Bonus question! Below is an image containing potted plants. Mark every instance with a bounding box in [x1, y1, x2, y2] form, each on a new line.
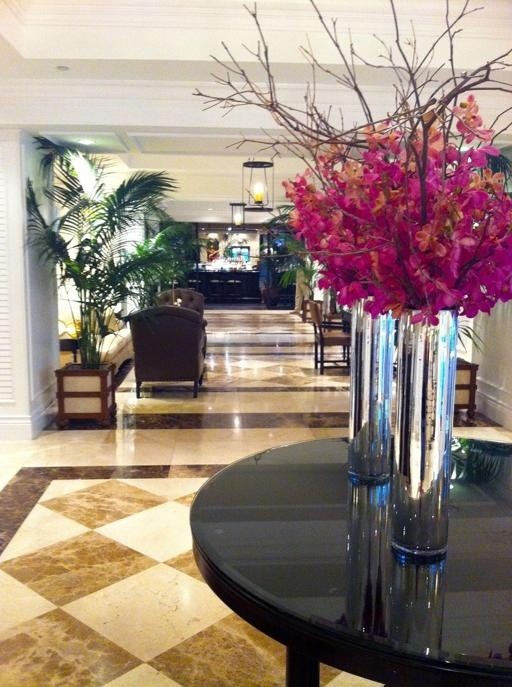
[22, 134, 207, 430]
[452, 356, 478, 420]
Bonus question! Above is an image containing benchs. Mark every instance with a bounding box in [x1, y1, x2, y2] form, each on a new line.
[69, 326, 131, 376]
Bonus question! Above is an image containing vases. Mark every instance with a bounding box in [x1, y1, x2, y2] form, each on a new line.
[347, 293, 459, 564]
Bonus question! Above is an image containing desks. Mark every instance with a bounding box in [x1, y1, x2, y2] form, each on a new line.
[191, 436, 509, 686]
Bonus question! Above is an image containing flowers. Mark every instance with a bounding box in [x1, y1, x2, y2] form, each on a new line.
[287, 96, 512, 321]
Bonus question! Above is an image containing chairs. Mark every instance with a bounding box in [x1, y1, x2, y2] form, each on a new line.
[306, 300, 353, 372]
[127, 289, 207, 399]
